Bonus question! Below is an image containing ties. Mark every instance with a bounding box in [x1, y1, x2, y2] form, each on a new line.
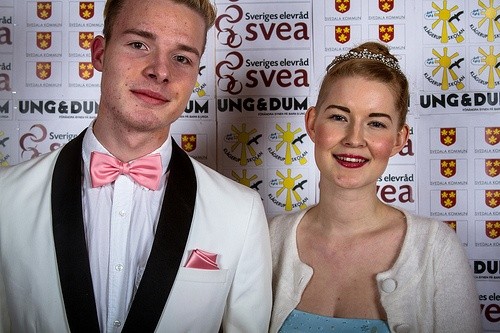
[89, 151, 162, 191]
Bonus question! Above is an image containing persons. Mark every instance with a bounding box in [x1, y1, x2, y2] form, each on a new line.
[261, 42, 481, 333]
[0, 0, 275, 333]
